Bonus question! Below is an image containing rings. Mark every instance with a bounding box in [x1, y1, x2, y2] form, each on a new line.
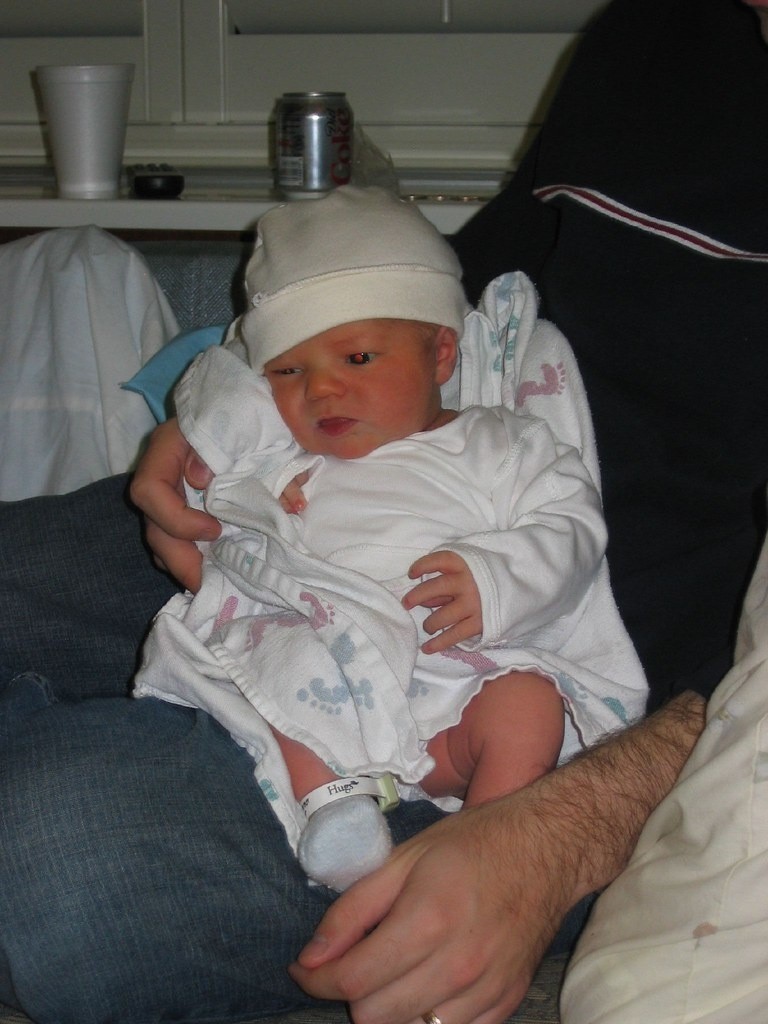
[421, 1011, 441, 1024]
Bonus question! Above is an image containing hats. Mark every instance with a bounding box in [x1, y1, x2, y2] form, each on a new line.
[240, 181, 469, 376]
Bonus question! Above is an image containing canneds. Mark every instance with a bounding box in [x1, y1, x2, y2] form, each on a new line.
[273, 91, 353, 200]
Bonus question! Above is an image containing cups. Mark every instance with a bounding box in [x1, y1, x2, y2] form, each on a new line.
[30, 61, 132, 200]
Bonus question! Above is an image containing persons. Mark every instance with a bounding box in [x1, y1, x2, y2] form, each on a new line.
[134, 181, 650, 886]
[0, 0, 768, 1024]
